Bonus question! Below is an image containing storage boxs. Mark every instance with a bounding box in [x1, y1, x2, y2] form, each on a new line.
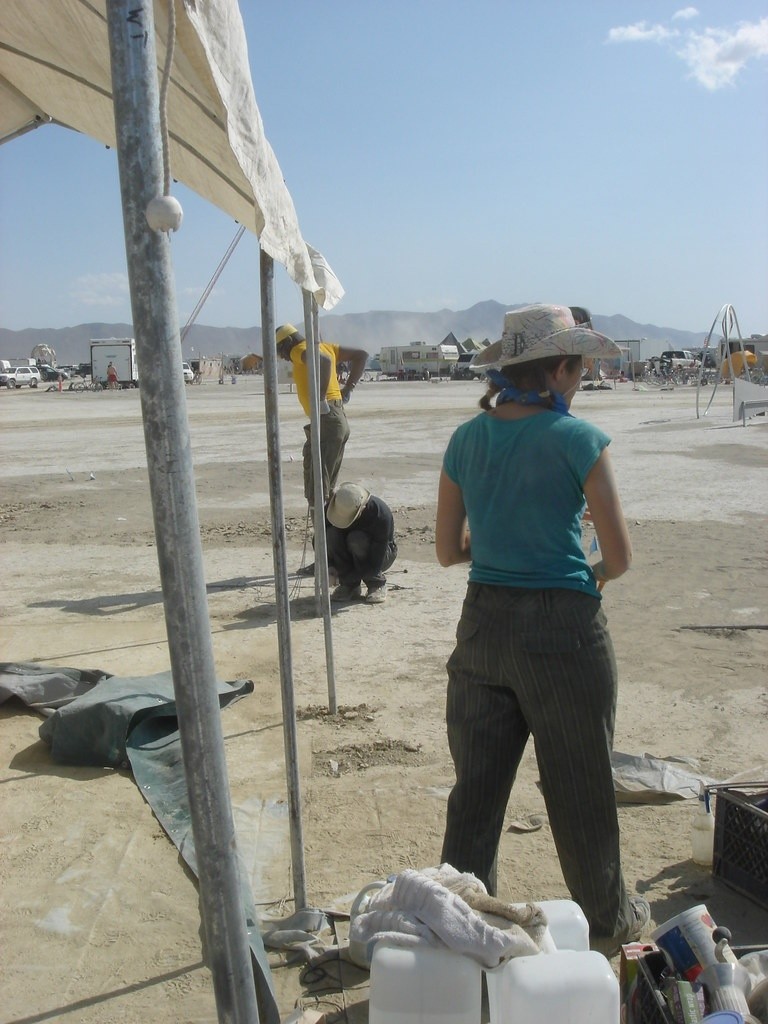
[712, 787, 768, 911]
[620, 943, 768, 1024]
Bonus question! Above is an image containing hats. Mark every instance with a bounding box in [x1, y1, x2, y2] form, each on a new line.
[469, 303, 626, 371]
[327, 481, 371, 528]
[275, 323, 302, 350]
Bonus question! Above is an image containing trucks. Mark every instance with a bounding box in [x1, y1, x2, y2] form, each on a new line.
[380, 341, 459, 381]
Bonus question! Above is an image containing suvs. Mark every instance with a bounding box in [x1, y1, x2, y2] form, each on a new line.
[182, 362, 193, 385]
[454, 353, 481, 380]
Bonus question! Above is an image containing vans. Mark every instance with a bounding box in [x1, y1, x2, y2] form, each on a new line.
[0, 367, 41, 389]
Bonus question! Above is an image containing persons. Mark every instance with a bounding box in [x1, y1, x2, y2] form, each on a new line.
[437, 306, 650, 959]
[311, 482, 398, 602]
[276, 323, 369, 576]
[425, 368, 429, 380]
[107, 364, 120, 391]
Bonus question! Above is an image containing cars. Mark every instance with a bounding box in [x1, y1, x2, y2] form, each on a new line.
[0, 358, 92, 382]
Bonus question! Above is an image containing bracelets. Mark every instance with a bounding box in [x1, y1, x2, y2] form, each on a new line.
[347, 379, 356, 387]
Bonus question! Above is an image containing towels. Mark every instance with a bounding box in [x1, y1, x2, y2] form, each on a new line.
[348, 859, 557, 972]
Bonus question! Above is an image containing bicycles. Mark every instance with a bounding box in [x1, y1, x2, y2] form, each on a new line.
[74, 375, 104, 393]
[194, 371, 202, 385]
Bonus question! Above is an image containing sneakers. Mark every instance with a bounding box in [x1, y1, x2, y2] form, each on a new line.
[331, 584, 362, 602]
[590, 893, 650, 958]
[366, 584, 388, 603]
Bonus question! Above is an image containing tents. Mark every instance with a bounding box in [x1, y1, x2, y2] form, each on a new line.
[0, 0, 346, 911]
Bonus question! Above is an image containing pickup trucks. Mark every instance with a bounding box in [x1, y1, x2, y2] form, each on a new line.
[654, 350, 702, 375]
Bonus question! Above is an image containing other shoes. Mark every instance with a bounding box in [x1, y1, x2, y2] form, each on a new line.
[329, 568, 340, 585]
[298, 564, 314, 576]
